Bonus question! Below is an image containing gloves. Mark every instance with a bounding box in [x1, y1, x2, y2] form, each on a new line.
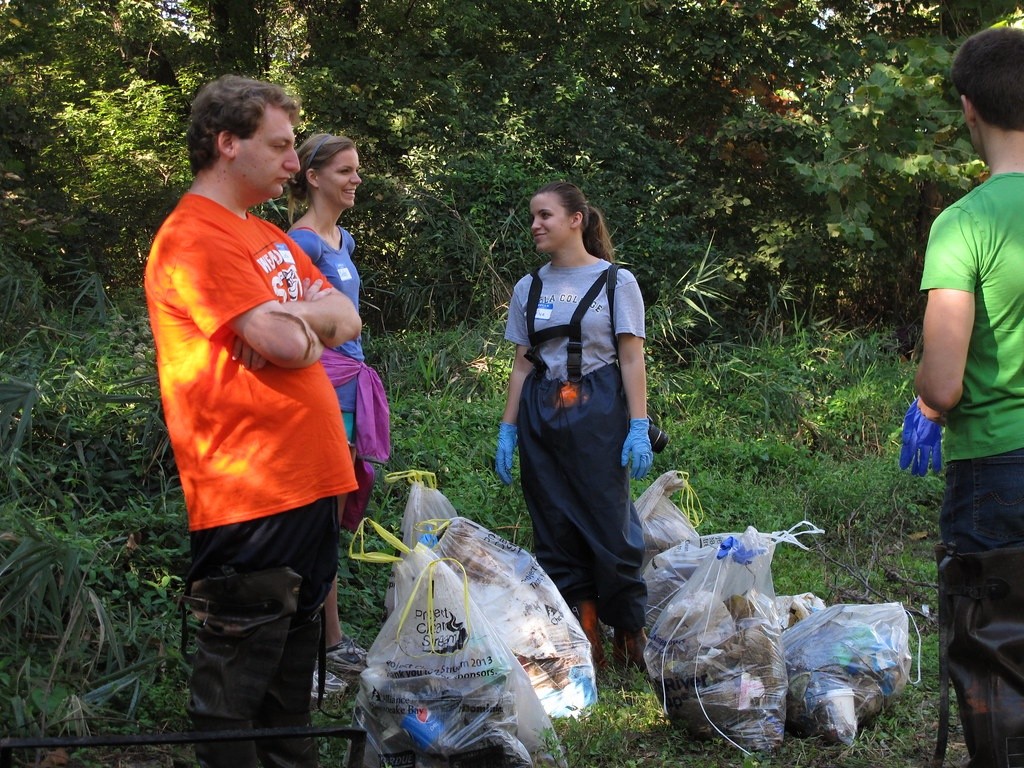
[621, 418, 653, 481]
[495, 422, 518, 485]
[900, 398, 943, 477]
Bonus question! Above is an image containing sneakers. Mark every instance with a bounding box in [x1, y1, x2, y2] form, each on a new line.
[310, 661, 349, 699]
[324, 634, 368, 671]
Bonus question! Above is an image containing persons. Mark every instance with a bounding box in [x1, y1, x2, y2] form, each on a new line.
[914, 28, 1024, 768]
[286, 133, 391, 699]
[144, 75, 363, 768]
[494, 182, 653, 679]
